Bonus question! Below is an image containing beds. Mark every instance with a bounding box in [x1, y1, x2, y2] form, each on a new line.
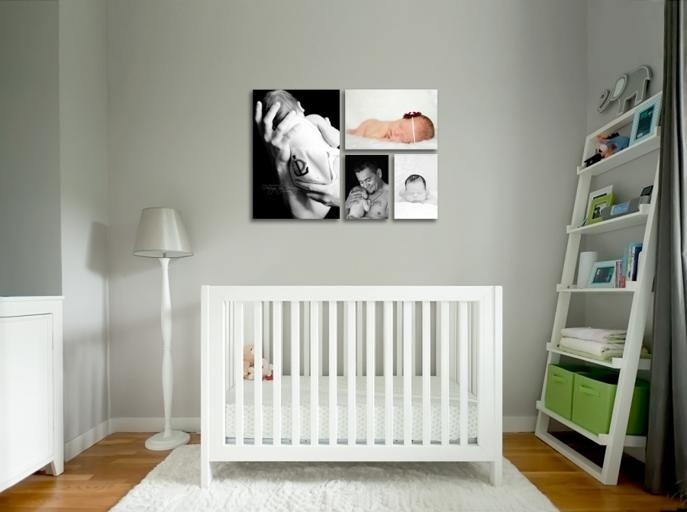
[200, 286, 504, 487]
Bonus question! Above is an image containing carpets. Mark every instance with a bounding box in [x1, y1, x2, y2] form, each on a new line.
[106, 442, 558, 512]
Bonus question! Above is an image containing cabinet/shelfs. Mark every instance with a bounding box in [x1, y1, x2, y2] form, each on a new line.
[533, 87, 662, 487]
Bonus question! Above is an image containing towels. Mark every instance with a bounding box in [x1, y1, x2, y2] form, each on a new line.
[559, 327, 652, 362]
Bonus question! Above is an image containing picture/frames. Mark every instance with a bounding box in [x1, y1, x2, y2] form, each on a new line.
[587, 261, 616, 285]
[626, 96, 662, 144]
[586, 185, 613, 219]
[585, 195, 612, 223]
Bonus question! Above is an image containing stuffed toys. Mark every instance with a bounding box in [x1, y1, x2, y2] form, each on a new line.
[245, 358, 272, 380]
[243, 342, 253, 377]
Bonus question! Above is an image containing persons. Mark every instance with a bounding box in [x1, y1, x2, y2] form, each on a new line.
[346, 112, 435, 144]
[254, 100, 339, 219]
[345, 187, 371, 219]
[395, 173, 437, 205]
[261, 90, 339, 191]
[344, 161, 389, 220]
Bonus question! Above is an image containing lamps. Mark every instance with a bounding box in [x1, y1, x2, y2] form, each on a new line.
[133, 206, 194, 451]
